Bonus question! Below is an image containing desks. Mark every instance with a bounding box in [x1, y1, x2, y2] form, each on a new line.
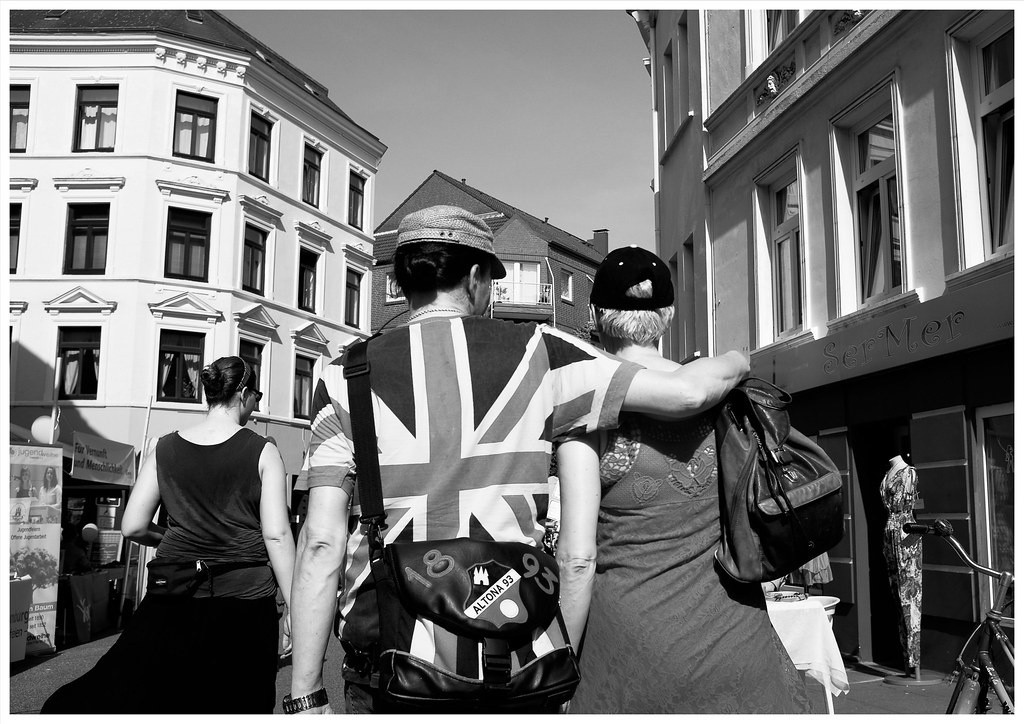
[765, 592, 851, 714]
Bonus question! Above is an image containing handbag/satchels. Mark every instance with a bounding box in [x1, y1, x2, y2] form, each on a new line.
[146, 558, 267, 597]
[342, 536, 582, 709]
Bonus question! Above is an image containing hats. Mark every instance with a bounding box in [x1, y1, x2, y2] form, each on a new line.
[590, 244, 675, 311]
[396, 205, 506, 280]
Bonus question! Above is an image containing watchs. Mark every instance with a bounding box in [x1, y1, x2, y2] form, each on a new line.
[283, 687, 329, 713]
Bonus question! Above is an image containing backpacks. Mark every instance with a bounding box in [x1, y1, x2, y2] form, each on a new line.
[714, 376, 846, 585]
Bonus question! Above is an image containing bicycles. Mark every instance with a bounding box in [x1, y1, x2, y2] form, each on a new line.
[903, 517, 1015, 714]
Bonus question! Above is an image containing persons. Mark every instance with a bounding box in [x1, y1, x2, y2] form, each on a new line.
[120, 355, 297, 714]
[879, 455, 924, 668]
[33, 467, 61, 510]
[290, 203, 750, 713]
[553, 246, 812, 714]
[14, 466, 38, 498]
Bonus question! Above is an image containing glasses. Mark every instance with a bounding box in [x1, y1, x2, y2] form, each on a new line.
[249, 388, 263, 402]
